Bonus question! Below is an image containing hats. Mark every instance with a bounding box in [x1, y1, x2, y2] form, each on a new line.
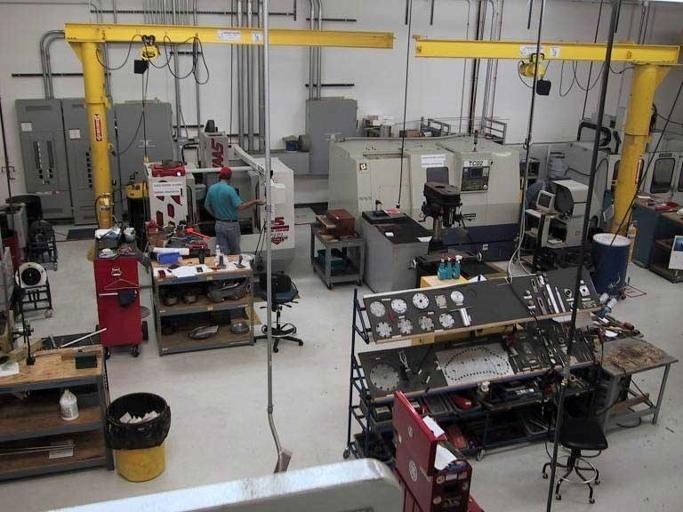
[220, 167, 232, 175]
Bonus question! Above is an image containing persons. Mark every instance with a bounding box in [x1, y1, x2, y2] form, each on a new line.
[205, 167, 266, 255]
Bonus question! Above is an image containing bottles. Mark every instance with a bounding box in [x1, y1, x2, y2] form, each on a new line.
[214, 244, 222, 257]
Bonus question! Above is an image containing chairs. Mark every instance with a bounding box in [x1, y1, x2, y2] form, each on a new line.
[540, 399, 608, 504]
[253, 273, 303, 353]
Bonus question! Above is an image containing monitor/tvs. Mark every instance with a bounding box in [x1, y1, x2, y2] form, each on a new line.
[537, 191, 554, 211]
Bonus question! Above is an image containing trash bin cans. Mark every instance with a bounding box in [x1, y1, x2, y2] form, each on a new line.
[105, 392, 169, 482]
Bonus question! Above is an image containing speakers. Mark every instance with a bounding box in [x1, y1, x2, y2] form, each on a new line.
[134, 60, 148, 74]
[537, 81, 550, 95]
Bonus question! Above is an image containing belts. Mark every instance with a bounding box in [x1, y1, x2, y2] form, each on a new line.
[216, 219, 238, 222]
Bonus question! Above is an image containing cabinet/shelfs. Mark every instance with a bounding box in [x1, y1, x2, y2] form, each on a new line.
[0, 344, 113, 483]
[146, 255, 252, 357]
